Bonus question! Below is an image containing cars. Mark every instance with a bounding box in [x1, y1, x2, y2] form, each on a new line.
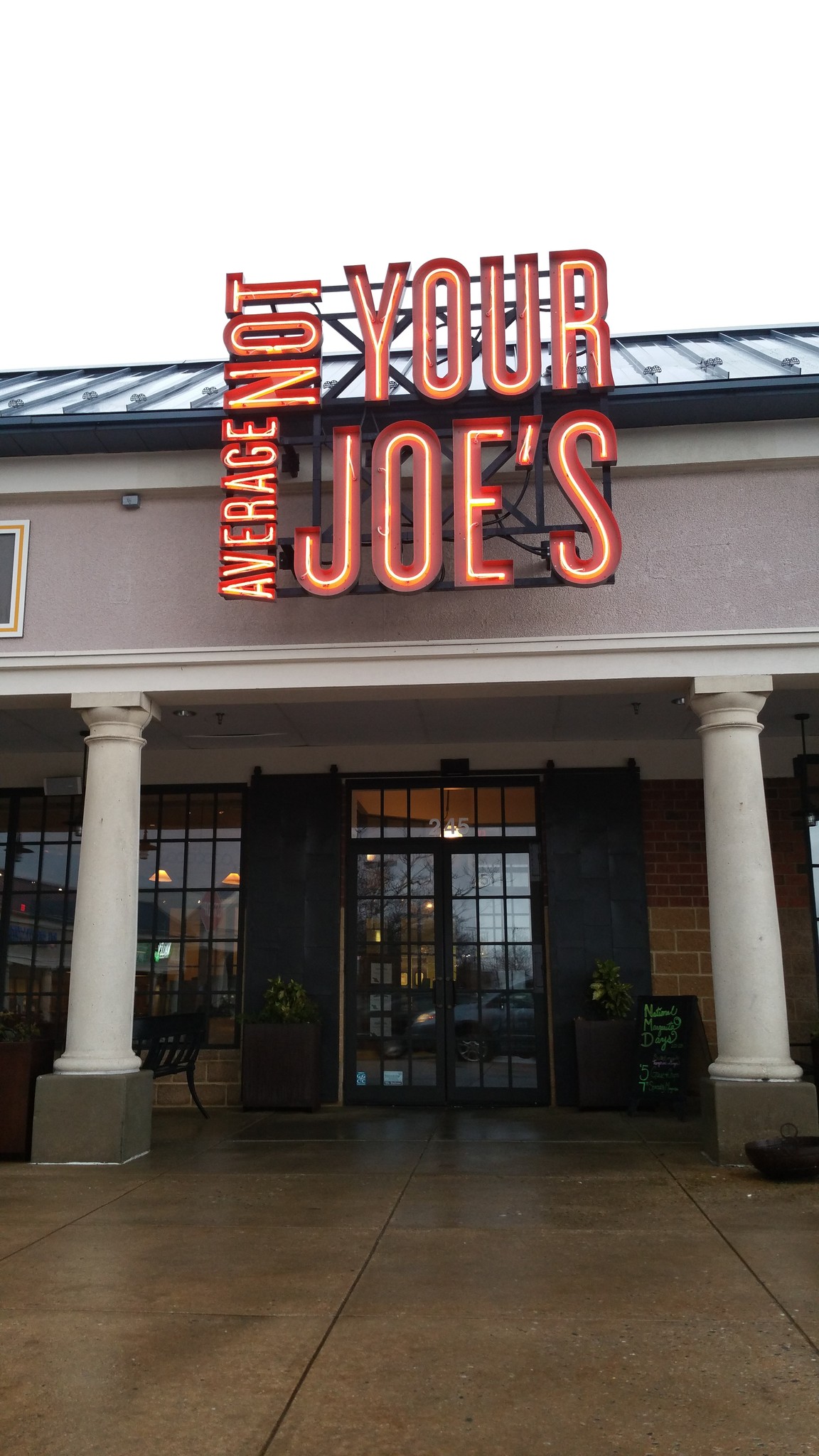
[402, 976, 536, 1063]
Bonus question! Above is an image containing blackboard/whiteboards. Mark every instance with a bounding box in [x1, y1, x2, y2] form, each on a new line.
[630, 995, 712, 1120]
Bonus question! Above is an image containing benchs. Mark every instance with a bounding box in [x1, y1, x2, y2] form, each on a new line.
[133, 1013, 210, 1121]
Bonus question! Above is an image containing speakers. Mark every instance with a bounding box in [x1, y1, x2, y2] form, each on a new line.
[440, 758, 469, 776]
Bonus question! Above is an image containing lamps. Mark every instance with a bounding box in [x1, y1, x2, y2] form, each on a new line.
[62, 730, 90, 836]
[440, 825, 462, 838]
[139, 806, 157, 859]
[222, 866, 240, 885]
[149, 870, 172, 882]
[791, 714, 819, 826]
[43, 775, 82, 796]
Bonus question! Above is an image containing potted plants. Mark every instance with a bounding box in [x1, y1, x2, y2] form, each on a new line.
[233, 976, 320, 1114]
[575, 958, 639, 1107]
[0, 1011, 55, 1161]
[208, 998, 235, 1045]
